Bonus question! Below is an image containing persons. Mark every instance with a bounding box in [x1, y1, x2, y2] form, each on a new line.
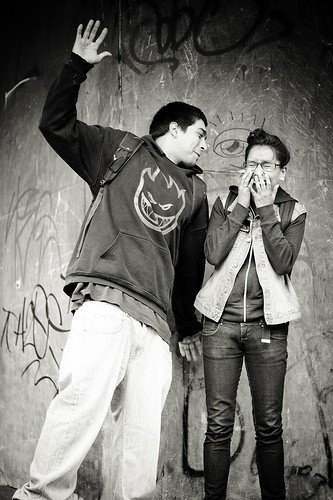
[11, 19, 208, 499]
[193, 129, 307, 500]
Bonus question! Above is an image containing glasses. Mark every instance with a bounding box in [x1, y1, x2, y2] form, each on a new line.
[242, 160, 282, 172]
